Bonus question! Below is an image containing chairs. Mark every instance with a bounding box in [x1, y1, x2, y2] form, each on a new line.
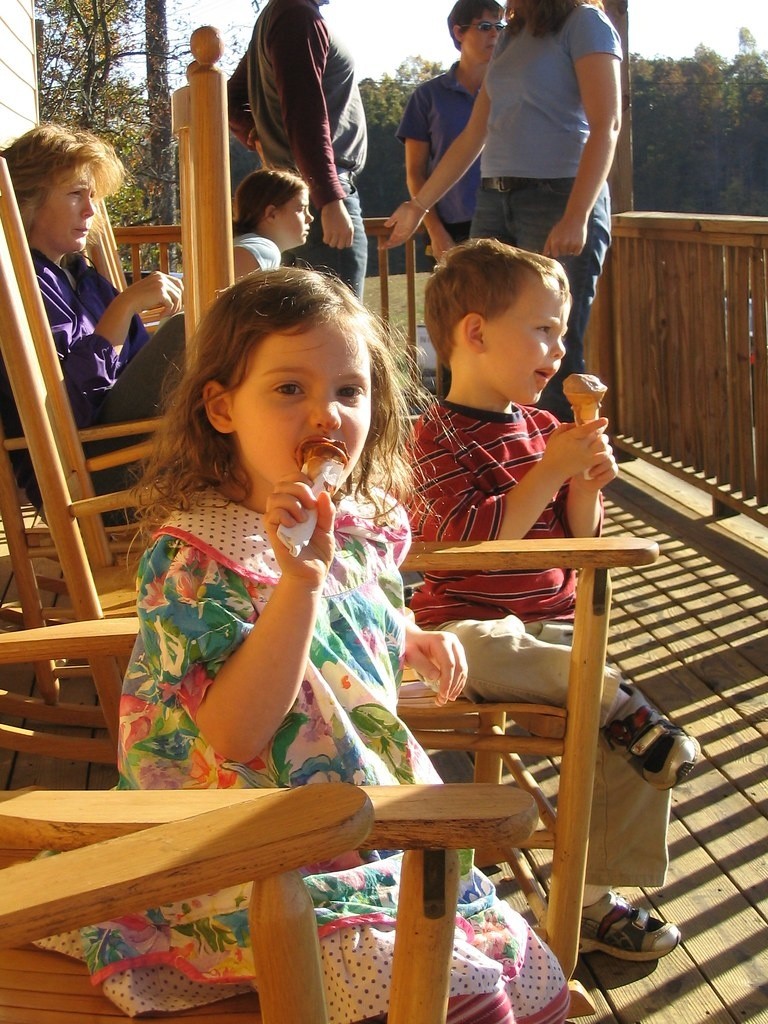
[1, 156, 658, 981]
[0, 782, 374, 1024]
[0, 617, 537, 1024]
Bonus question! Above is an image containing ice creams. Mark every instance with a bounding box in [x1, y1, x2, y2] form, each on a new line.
[301, 441, 349, 482]
[563, 374, 608, 481]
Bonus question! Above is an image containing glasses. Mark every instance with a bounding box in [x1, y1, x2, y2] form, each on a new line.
[459, 21, 505, 31]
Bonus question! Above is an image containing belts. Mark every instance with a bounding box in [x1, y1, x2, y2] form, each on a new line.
[336, 166, 353, 181]
[482, 178, 535, 193]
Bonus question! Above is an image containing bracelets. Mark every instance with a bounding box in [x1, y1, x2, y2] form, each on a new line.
[411, 194, 433, 215]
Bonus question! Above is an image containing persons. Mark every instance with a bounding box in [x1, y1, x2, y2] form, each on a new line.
[226, 0, 372, 299]
[399, 234, 700, 963]
[1, 121, 188, 531]
[117, 267, 573, 1024]
[376, 0, 623, 421]
[232, 165, 314, 279]
[392, 0, 506, 261]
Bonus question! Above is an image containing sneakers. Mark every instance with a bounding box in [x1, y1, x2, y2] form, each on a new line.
[579, 890, 681, 961]
[599, 683, 701, 791]
